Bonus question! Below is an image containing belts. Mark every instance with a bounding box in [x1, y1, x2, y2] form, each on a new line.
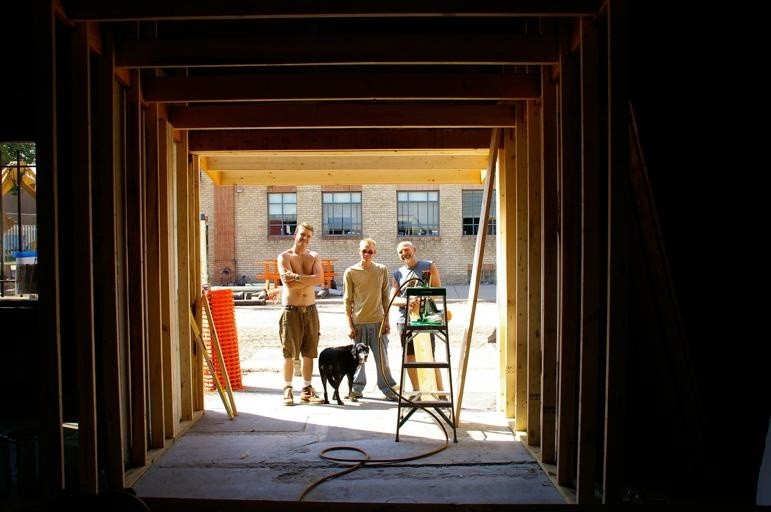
[284, 305, 316, 315]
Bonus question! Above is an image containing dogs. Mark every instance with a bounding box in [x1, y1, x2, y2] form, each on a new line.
[318, 342, 370, 405]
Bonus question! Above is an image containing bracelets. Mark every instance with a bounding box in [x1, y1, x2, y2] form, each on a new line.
[384, 312, 389, 316]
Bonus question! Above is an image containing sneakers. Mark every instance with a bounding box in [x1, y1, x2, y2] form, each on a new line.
[386, 395, 398, 401]
[438, 396, 448, 401]
[345, 393, 362, 399]
[409, 393, 421, 401]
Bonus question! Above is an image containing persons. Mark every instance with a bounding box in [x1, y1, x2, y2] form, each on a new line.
[276, 221, 327, 406]
[339, 237, 398, 402]
[389, 241, 449, 401]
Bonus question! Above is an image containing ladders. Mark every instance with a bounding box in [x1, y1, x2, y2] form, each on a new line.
[396, 288, 458, 442]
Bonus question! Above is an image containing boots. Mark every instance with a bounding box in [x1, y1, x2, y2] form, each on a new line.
[283, 386, 294, 406]
[302, 385, 324, 403]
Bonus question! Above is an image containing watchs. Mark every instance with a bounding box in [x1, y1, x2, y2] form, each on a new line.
[295, 274, 300, 282]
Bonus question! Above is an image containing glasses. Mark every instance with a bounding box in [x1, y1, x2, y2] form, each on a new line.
[362, 249, 374, 256]
